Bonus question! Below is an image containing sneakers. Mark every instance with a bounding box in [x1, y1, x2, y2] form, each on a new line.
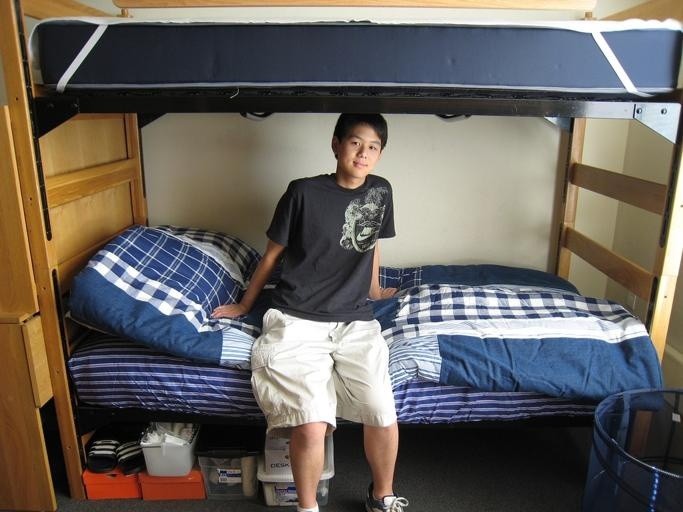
[364, 480, 411, 512]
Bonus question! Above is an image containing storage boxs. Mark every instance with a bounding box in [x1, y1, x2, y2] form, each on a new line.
[83, 423, 336, 508]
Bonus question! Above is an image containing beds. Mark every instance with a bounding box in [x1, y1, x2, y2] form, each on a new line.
[0, 0, 683, 512]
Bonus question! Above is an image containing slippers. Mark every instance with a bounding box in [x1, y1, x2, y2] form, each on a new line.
[115, 434, 146, 475]
[86, 435, 117, 473]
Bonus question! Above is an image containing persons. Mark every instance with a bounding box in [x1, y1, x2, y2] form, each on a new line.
[209, 112, 410, 512]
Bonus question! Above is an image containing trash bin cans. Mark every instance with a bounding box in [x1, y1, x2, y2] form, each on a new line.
[581, 389, 683, 512]
